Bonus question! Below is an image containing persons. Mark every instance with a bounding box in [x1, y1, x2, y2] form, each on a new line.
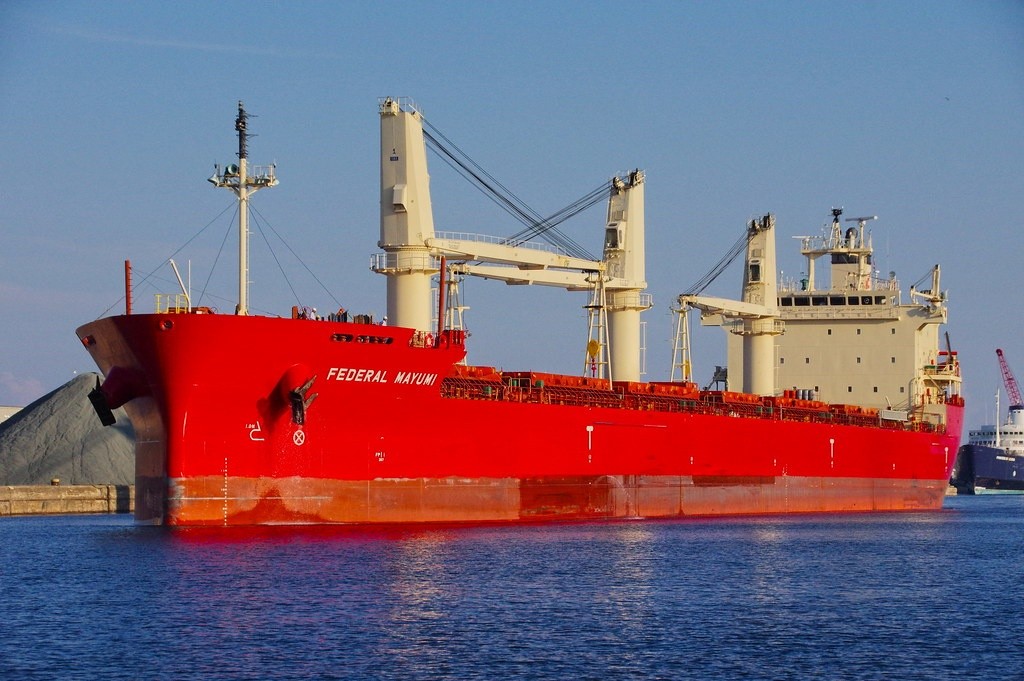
[378, 317, 388, 343]
[310, 308, 316, 321]
[302, 307, 307, 320]
[336, 309, 344, 317]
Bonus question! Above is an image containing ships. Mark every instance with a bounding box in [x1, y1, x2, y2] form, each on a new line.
[954, 346, 1023, 495]
[68, 89, 967, 521]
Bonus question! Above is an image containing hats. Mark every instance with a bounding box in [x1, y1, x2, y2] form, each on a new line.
[312, 308, 316, 310]
[382, 316, 387, 320]
